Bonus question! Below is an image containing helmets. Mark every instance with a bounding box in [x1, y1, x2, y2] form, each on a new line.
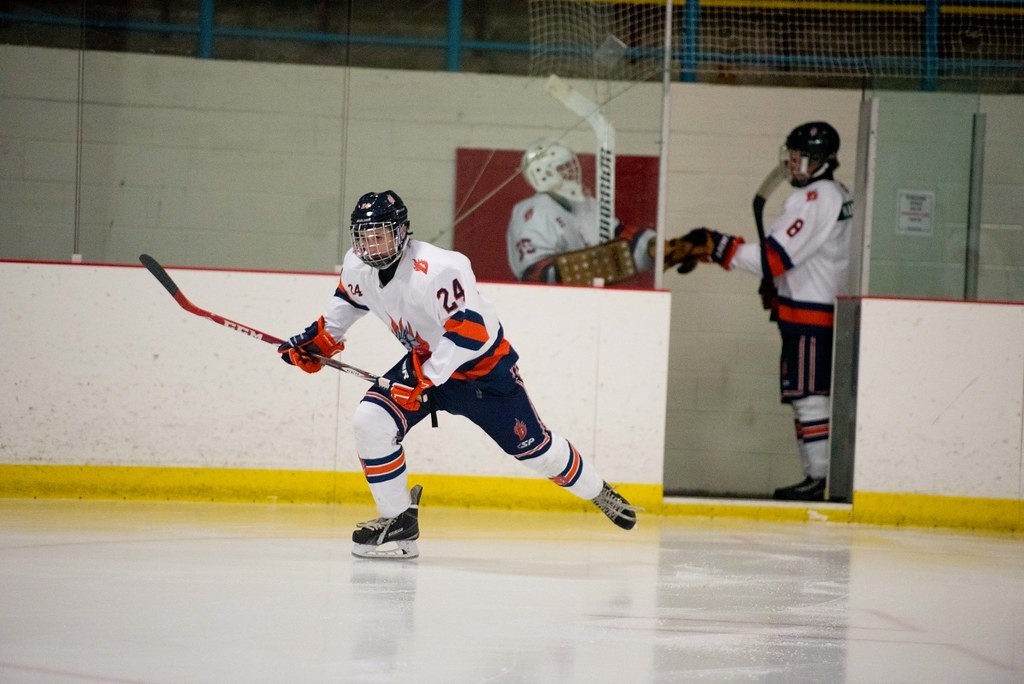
[785, 121, 840, 189]
[520, 137, 585, 202]
[350, 190, 409, 267]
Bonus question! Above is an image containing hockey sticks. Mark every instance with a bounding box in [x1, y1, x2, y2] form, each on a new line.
[750, 158, 788, 333]
[136, 250, 433, 407]
[544, 68, 619, 288]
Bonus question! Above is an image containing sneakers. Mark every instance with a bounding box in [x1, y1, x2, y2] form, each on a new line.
[774, 475, 813, 500]
[794, 477, 826, 501]
[589, 480, 644, 531]
[352, 485, 423, 558]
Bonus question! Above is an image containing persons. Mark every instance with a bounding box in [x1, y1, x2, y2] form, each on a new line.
[507, 139, 678, 288]
[279, 190, 636, 559]
[668, 120, 854, 502]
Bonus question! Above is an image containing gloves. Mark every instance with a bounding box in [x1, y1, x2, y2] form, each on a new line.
[277, 316, 345, 373]
[649, 228, 746, 274]
[384, 349, 436, 411]
